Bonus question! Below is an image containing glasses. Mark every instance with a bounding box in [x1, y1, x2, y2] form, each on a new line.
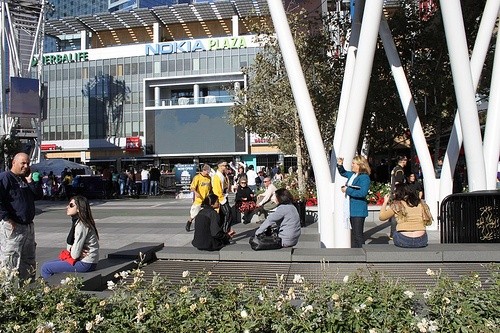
[263, 180, 270, 183]
[222, 165, 229, 167]
[69, 203, 76, 207]
[241, 182, 247, 184]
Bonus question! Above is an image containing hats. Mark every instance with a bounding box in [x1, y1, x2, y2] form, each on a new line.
[398, 155, 408, 161]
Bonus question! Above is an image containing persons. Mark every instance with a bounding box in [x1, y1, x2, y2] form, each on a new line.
[273, 171, 284, 182]
[408, 170, 424, 199]
[246, 164, 257, 185]
[186, 162, 211, 233]
[140, 166, 150, 195]
[254, 188, 301, 247]
[40, 196, 100, 277]
[190, 194, 235, 251]
[235, 178, 257, 224]
[211, 159, 233, 234]
[254, 177, 280, 213]
[26, 171, 74, 200]
[150, 166, 160, 195]
[435, 156, 462, 226]
[388, 155, 408, 240]
[102, 164, 161, 197]
[186, 155, 435, 251]
[379, 183, 434, 247]
[0, 152, 37, 283]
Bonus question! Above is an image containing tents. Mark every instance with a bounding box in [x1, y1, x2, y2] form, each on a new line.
[29, 159, 90, 175]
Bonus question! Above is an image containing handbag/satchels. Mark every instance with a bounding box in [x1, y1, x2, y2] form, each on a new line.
[248, 227, 282, 251]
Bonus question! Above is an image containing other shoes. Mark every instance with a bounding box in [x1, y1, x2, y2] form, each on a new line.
[186, 220, 192, 232]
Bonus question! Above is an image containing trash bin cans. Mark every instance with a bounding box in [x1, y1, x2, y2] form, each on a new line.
[292, 199, 308, 227]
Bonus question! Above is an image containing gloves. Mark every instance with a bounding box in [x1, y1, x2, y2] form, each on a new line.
[59, 249, 76, 266]
